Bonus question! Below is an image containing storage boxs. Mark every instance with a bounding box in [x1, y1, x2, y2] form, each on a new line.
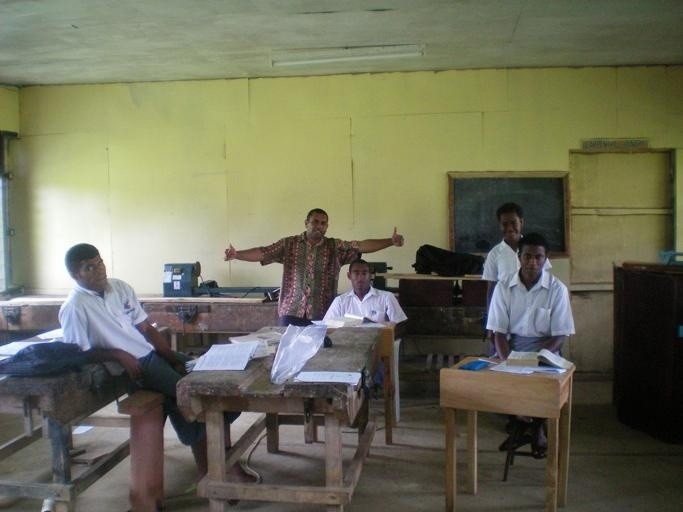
[398, 279, 453, 306]
[461, 280, 488, 307]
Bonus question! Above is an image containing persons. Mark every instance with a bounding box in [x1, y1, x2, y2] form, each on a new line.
[481, 199, 553, 355]
[222, 208, 405, 326]
[322, 259, 412, 388]
[58, 242, 255, 507]
[485, 231, 577, 460]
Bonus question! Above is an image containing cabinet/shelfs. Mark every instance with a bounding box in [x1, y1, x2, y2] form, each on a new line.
[610, 260, 683, 447]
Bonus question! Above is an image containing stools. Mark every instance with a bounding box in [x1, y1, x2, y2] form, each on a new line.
[501, 411, 547, 482]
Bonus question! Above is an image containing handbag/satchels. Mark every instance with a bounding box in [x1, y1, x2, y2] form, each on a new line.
[0, 342, 89, 376]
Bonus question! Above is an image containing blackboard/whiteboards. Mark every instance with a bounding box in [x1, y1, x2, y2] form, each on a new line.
[447, 171, 573, 259]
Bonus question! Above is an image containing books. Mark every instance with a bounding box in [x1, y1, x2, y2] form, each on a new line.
[183, 329, 286, 373]
[506, 348, 573, 369]
[324, 313, 378, 328]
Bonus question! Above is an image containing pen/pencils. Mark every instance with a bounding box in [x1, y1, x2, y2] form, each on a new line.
[371, 311, 376, 317]
[478, 358, 498, 365]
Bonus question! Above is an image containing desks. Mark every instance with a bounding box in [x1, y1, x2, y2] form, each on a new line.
[441, 354, 575, 511]
[173, 325, 384, 512]
[0, 325, 171, 512]
[1, 289, 496, 354]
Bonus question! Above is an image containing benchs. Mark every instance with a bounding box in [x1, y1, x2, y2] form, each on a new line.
[120, 388, 170, 511]
